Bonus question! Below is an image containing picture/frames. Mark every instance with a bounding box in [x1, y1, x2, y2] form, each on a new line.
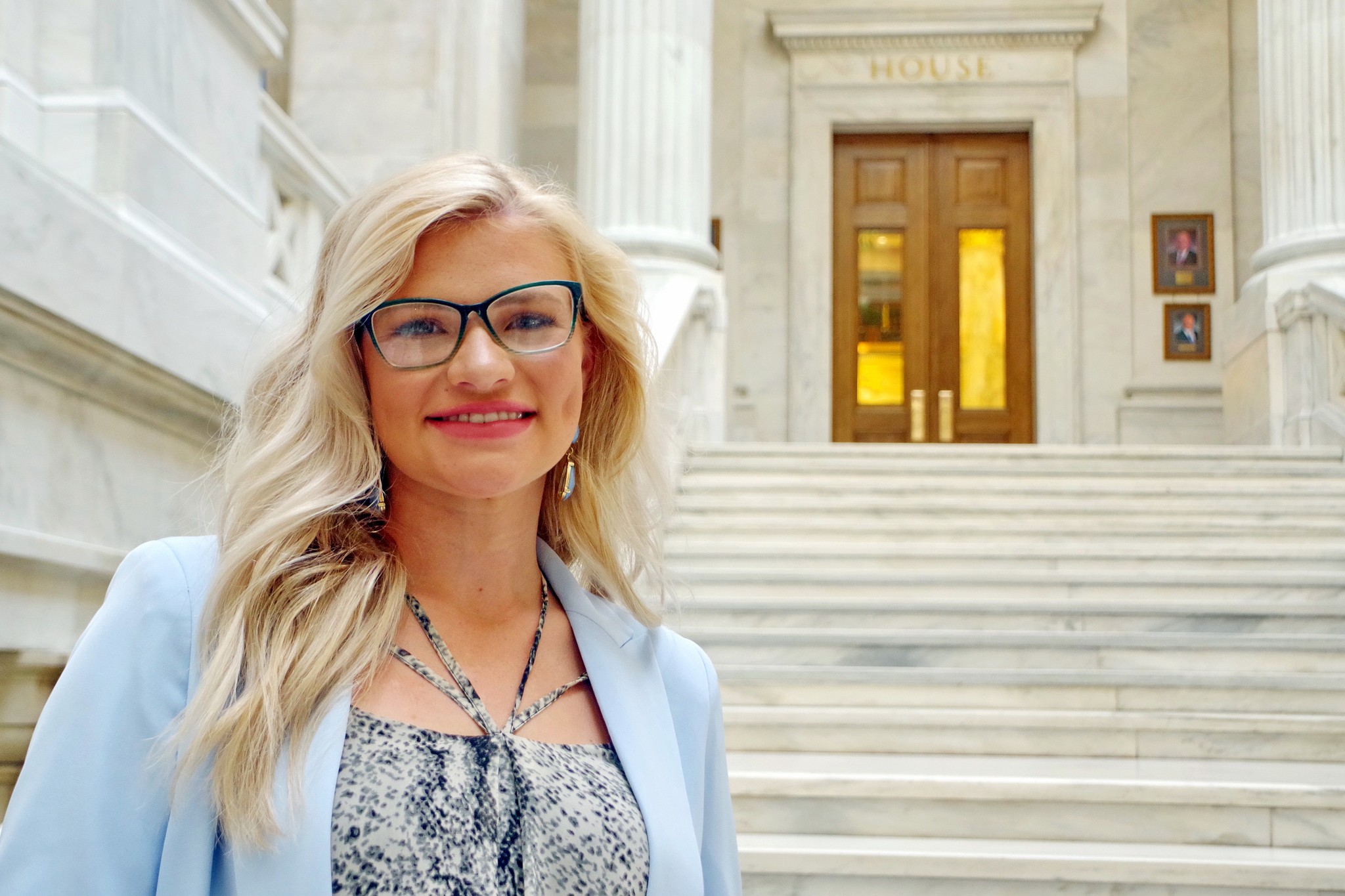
[1152, 215, 1216, 295]
[1164, 303, 1211, 359]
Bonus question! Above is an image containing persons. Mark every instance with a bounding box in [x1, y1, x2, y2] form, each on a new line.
[1167, 227, 1198, 266]
[0, 160, 744, 896]
[1171, 308, 1201, 344]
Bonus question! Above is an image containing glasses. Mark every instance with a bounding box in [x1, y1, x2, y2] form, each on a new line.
[355, 281, 587, 371]
